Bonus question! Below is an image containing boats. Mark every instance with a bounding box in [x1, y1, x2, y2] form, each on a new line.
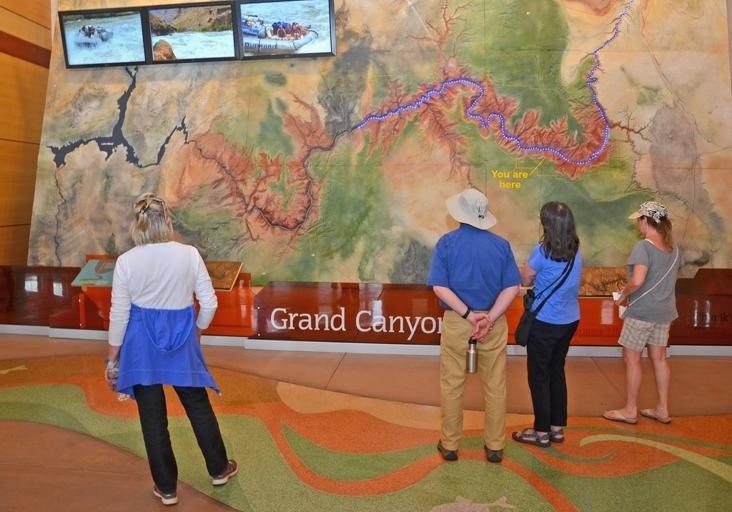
[242, 13, 320, 54]
[72, 27, 114, 49]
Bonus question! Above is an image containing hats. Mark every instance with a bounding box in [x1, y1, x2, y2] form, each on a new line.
[444, 187, 498, 231]
[628, 200, 669, 221]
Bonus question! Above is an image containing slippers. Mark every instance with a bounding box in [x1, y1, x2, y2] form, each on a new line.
[603, 410, 640, 425]
[639, 407, 673, 425]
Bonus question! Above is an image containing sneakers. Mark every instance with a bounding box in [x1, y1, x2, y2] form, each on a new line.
[152, 482, 178, 506]
[484, 444, 504, 463]
[211, 457, 239, 486]
[437, 439, 459, 461]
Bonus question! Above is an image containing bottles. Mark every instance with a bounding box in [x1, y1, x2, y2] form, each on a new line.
[465, 336, 478, 373]
[106, 359, 131, 402]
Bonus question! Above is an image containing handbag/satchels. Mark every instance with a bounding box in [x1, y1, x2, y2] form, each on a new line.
[612, 281, 629, 320]
[514, 308, 535, 348]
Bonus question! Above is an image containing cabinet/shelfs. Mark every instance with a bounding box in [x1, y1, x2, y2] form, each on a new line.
[669, 267, 732, 346]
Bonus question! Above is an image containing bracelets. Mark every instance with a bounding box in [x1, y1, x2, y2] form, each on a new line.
[462, 307, 471, 319]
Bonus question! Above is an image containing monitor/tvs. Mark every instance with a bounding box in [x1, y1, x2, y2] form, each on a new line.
[233, 0, 336, 60]
[58, 7, 150, 68]
[145, 1, 239, 63]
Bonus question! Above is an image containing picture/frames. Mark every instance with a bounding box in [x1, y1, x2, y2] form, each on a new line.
[58, 0, 336, 68]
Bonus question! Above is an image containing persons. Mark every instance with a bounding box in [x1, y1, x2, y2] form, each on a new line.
[424, 189, 521, 462]
[511, 202, 582, 447]
[104, 193, 238, 506]
[601, 202, 682, 425]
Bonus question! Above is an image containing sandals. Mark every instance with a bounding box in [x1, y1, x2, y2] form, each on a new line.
[511, 427, 565, 448]
